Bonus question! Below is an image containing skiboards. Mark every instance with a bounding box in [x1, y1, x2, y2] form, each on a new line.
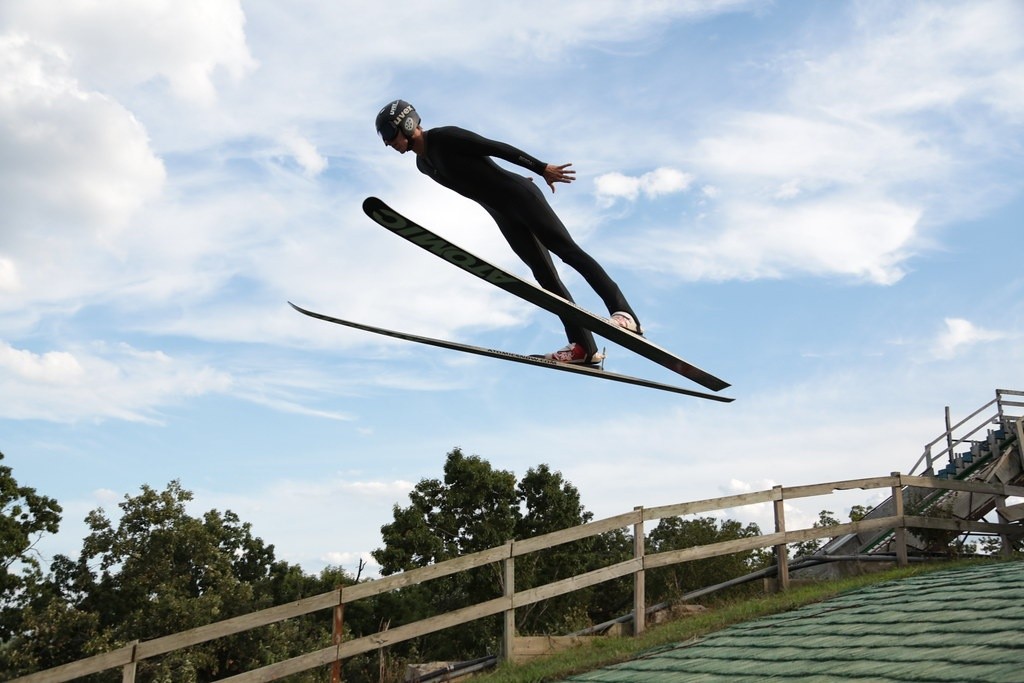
[287, 195, 737, 405]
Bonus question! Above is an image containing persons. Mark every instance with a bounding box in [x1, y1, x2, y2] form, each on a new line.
[375, 98, 645, 365]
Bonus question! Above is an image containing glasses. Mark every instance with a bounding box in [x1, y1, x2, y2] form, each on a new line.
[381, 123, 399, 145]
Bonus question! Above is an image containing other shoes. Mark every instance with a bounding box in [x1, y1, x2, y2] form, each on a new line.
[542, 342, 601, 363]
[602, 312, 643, 334]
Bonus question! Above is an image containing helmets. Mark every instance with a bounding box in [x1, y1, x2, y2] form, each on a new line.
[376, 99, 422, 137]
